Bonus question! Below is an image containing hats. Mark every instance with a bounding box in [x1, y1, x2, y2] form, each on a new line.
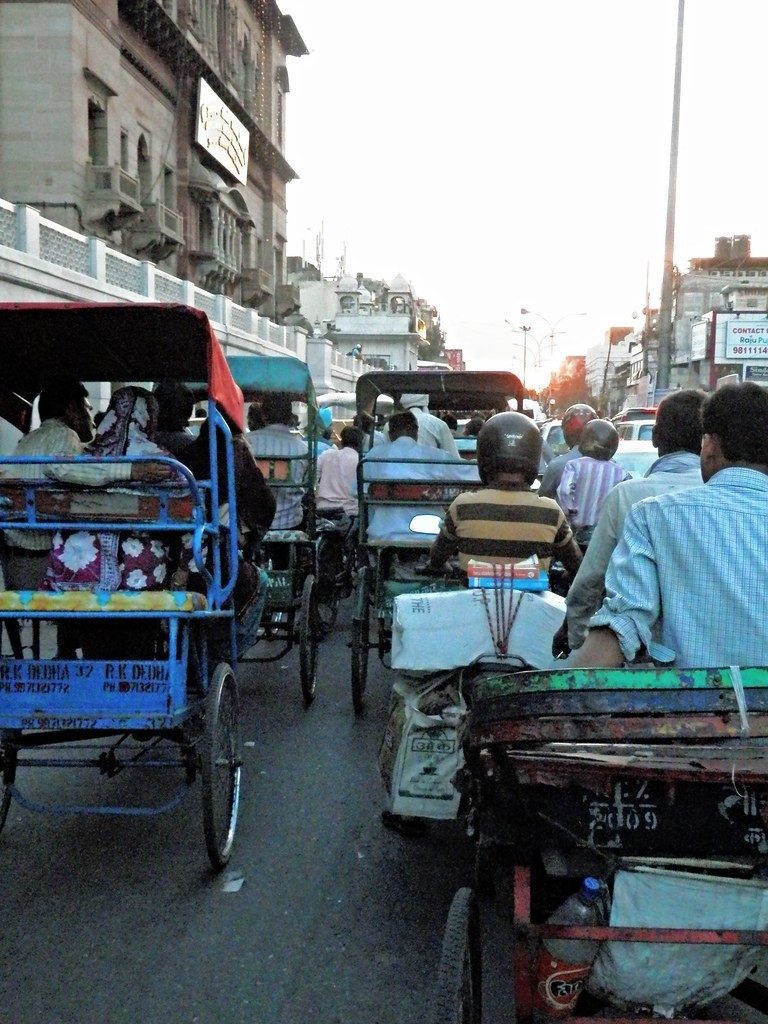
[401, 393, 429, 410]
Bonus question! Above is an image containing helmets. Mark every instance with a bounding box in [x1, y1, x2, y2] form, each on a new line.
[477, 411, 543, 485]
[561, 404, 599, 449]
[579, 418, 619, 461]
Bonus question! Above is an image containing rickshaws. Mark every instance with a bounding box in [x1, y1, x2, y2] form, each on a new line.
[436, 664, 768, 1024]
[0, 300, 348, 869]
[354, 369, 556, 713]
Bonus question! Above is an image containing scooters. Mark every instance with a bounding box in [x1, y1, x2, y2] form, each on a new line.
[406, 512, 598, 898]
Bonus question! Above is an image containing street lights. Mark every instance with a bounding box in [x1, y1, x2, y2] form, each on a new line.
[510, 308, 589, 374]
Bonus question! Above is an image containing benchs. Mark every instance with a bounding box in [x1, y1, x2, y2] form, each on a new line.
[355, 458, 483, 550]
[251, 452, 308, 543]
[0, 455, 213, 611]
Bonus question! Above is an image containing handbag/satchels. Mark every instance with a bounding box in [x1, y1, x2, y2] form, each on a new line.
[378, 669, 466, 820]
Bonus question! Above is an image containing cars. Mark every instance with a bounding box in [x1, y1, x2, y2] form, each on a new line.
[515, 399, 658, 486]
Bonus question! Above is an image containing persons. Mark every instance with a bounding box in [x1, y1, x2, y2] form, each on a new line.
[363, 411, 477, 566]
[564, 387, 707, 649]
[154, 379, 276, 591]
[383, 394, 460, 459]
[0, 379, 171, 661]
[538, 403, 598, 496]
[572, 380, 767, 671]
[45, 387, 205, 660]
[245, 397, 308, 570]
[462, 419, 483, 436]
[306, 414, 385, 574]
[430, 410, 584, 598]
[443, 414, 463, 438]
[559, 420, 634, 543]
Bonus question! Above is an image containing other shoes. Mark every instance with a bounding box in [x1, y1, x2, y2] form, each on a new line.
[379, 812, 426, 838]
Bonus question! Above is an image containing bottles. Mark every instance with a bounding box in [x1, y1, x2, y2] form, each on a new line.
[530, 877, 604, 1024]
